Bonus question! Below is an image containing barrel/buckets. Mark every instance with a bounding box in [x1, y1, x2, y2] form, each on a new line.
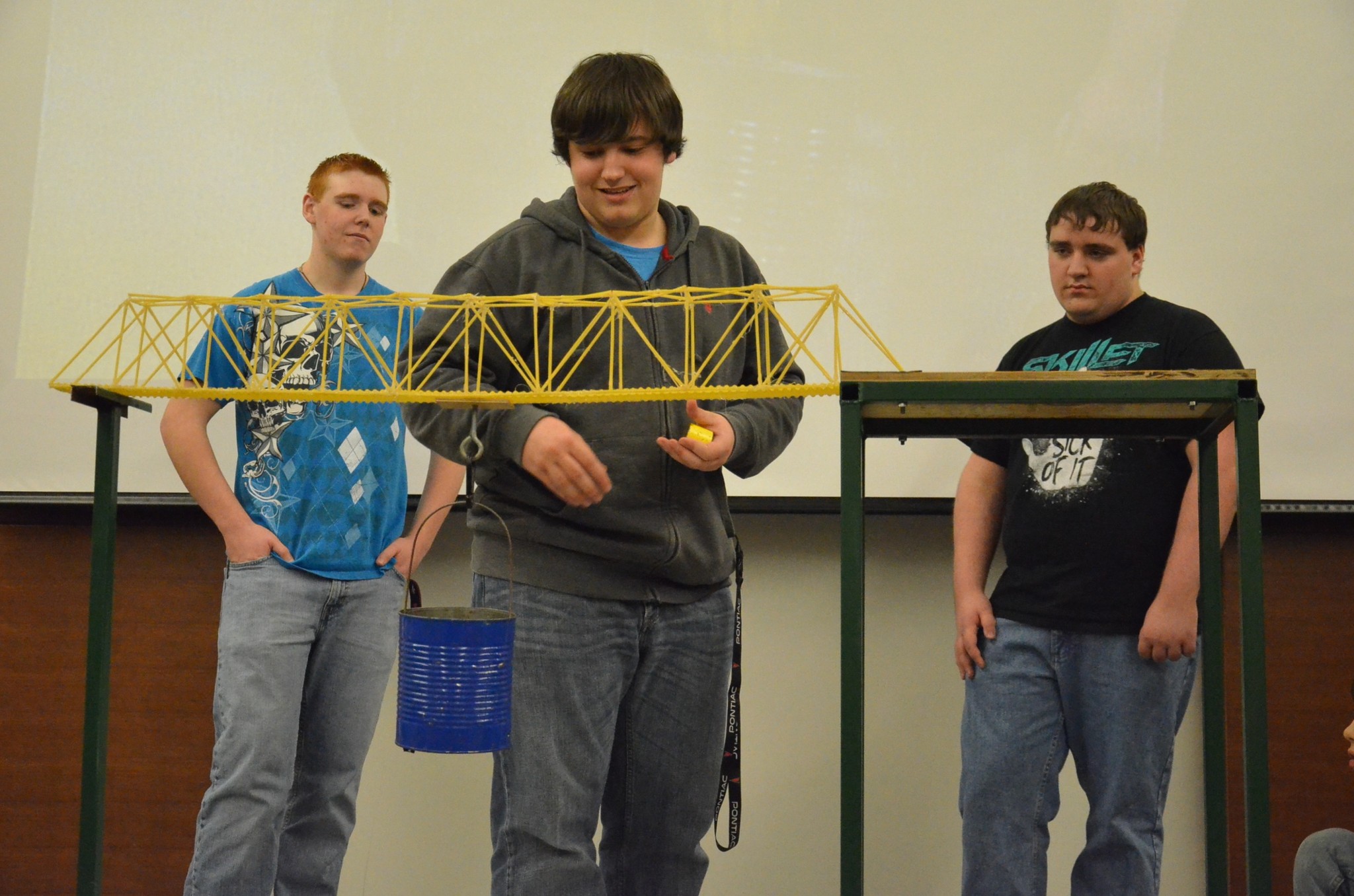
[394, 501, 517, 754]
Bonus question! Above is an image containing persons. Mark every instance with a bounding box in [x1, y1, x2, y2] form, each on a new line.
[953, 182, 1264, 896]
[1293, 719, 1354, 896]
[396, 55, 805, 896]
[160, 154, 466, 896]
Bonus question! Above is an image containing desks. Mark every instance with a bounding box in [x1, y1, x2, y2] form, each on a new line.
[835, 370, 1273, 896]
